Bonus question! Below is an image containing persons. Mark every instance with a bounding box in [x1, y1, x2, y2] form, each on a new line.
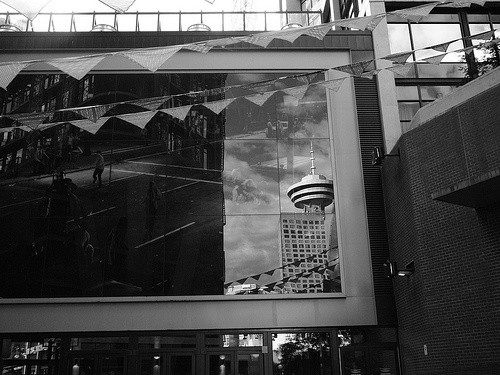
[264, 111, 315, 141]
[142, 180, 162, 242]
[17, 213, 131, 296]
[90, 150, 107, 187]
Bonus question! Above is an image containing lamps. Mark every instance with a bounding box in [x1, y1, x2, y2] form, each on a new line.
[371, 146, 400, 168]
[382, 261, 415, 279]
